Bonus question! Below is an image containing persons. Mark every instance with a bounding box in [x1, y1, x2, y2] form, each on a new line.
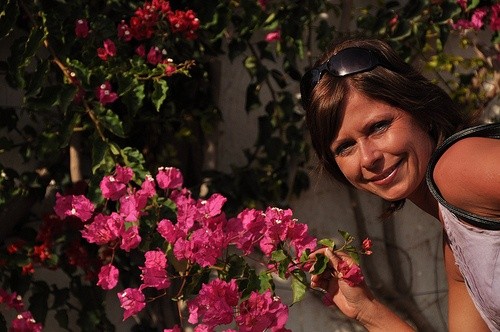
[300, 40, 500, 332]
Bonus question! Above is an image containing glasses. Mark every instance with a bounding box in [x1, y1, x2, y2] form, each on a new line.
[300, 46, 398, 109]
